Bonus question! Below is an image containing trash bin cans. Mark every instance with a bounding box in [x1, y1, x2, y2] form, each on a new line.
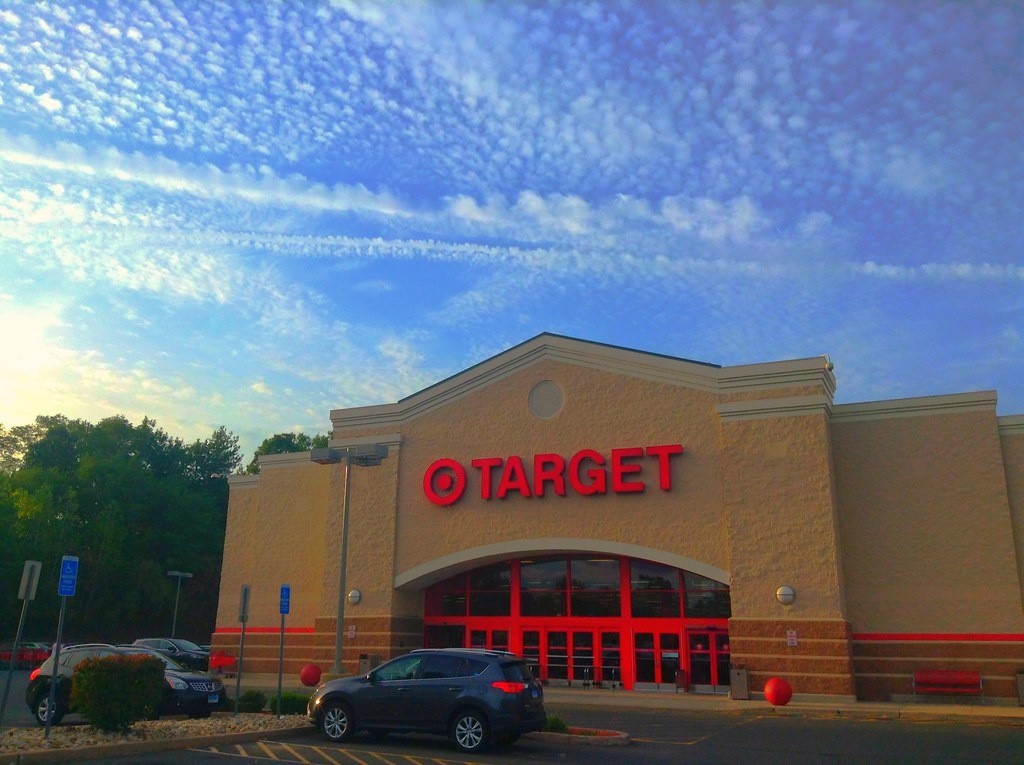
[728, 662, 751, 701]
[356, 653, 379, 675]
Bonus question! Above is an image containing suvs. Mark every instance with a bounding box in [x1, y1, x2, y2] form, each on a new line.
[133, 637, 210, 673]
[307, 648, 548, 755]
[25, 643, 228, 727]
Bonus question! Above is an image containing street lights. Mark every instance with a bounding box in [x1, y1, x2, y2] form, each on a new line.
[167, 571, 193, 639]
[310, 442, 391, 674]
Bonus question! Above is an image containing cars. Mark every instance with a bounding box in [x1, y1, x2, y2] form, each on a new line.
[0, 642, 52, 669]
